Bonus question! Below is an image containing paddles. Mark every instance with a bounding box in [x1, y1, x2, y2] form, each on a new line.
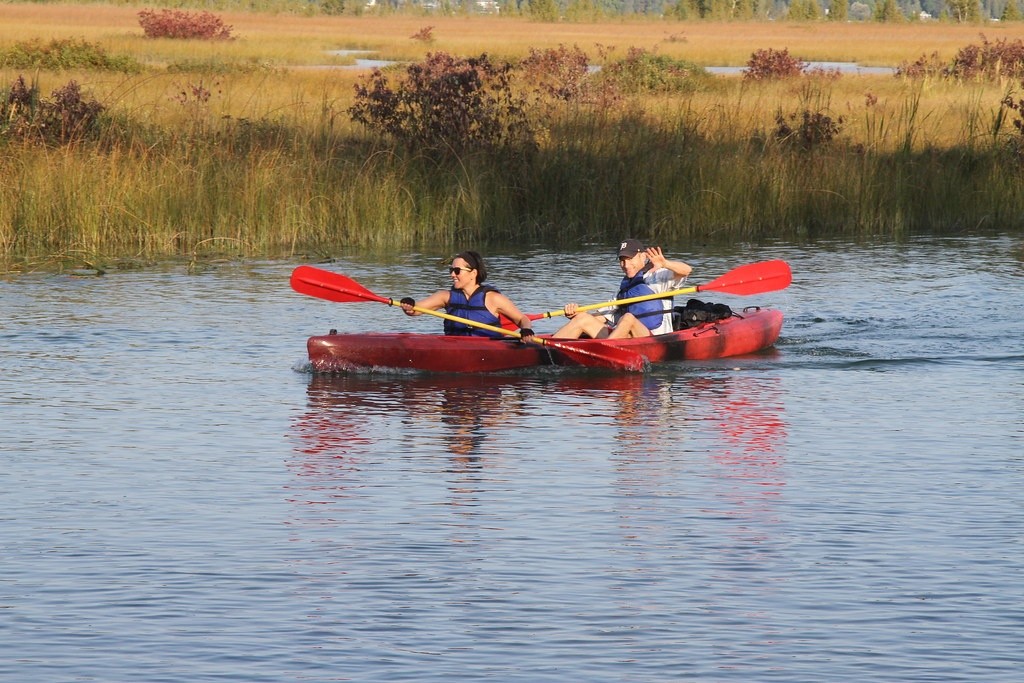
[498, 260, 792, 335]
[289, 265, 643, 374]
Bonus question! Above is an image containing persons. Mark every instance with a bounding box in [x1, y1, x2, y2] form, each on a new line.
[400, 251, 535, 344]
[552, 238, 691, 339]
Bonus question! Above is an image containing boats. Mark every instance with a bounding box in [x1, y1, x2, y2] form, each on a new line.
[307, 306, 782, 376]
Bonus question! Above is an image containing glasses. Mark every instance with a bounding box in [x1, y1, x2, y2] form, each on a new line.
[449, 267, 472, 275]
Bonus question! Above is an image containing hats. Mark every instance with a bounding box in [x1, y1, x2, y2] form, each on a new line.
[616, 239, 647, 260]
